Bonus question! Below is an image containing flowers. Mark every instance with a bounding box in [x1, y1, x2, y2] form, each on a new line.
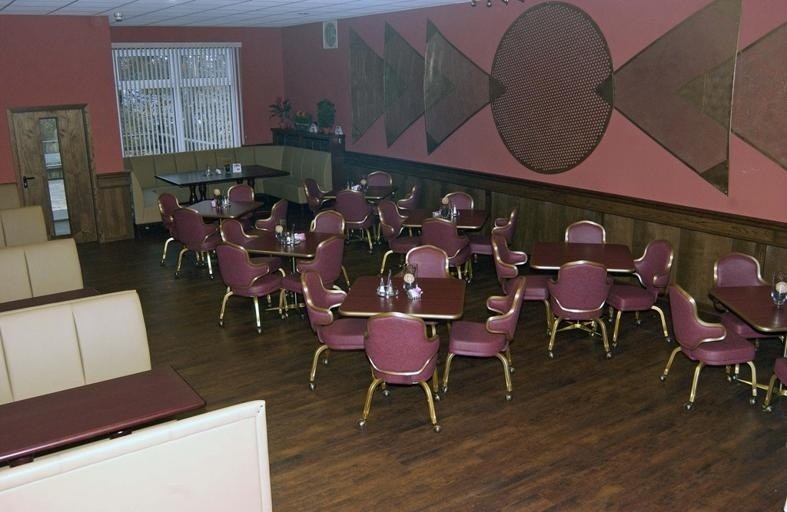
[270, 97, 292, 122]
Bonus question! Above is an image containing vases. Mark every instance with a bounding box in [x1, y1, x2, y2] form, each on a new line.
[278, 122, 288, 129]
[296, 123, 310, 131]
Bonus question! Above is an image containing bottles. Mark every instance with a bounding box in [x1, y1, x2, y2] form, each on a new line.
[274, 230, 302, 247]
[211, 195, 233, 210]
[375, 268, 399, 298]
[201, 160, 231, 177]
[431, 202, 460, 221]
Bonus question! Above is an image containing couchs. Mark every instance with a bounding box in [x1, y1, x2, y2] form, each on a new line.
[124, 145, 333, 239]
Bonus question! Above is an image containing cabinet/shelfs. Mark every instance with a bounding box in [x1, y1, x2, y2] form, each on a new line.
[270, 128, 345, 168]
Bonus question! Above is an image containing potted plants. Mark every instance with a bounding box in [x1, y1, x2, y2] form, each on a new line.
[317, 100, 335, 134]
[295, 111, 311, 123]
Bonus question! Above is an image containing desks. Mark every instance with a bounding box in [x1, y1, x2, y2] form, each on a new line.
[155, 164, 289, 205]
